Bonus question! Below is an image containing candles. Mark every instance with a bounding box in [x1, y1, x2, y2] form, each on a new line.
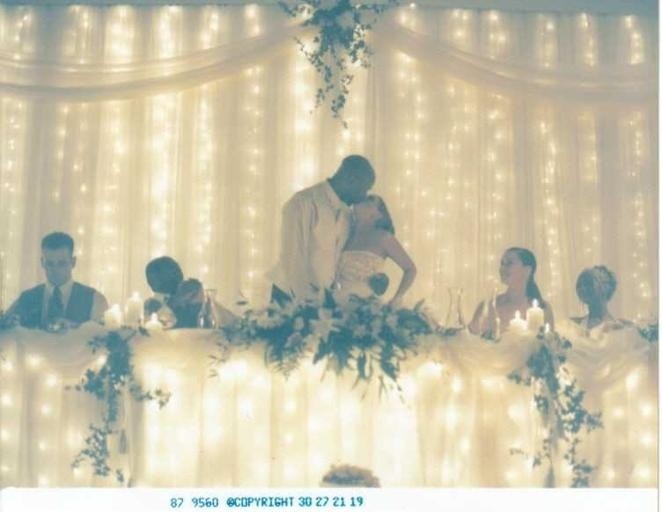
[146, 312, 166, 334]
[524, 299, 546, 336]
[510, 310, 530, 340]
[127, 291, 145, 327]
[103, 305, 125, 331]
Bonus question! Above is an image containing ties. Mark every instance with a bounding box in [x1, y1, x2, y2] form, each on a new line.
[45, 286, 62, 328]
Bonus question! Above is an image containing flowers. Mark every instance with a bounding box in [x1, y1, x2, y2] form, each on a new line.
[201, 278, 442, 406]
[0, 305, 26, 339]
[640, 320, 657, 344]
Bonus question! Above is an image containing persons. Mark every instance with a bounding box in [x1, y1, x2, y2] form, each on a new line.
[5, 233, 108, 329]
[268, 156, 375, 316]
[571, 267, 635, 334]
[468, 247, 555, 340]
[338, 195, 416, 310]
[145, 256, 204, 328]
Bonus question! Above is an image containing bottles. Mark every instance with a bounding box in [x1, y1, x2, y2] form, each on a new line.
[445, 286, 465, 327]
[482, 286, 500, 344]
[197, 288, 217, 329]
